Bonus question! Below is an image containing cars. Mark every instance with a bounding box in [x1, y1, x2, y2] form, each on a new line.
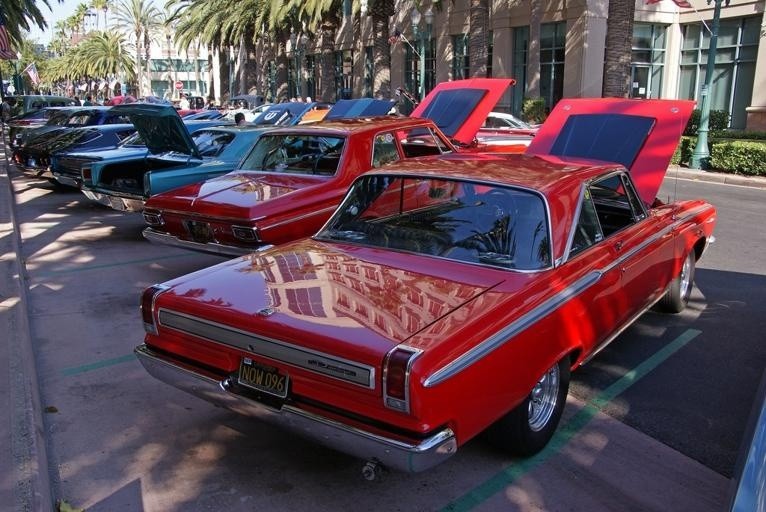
[0, 93, 400, 214]
[133, 96, 717, 483]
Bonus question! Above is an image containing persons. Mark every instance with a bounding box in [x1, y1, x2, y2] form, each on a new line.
[234, 113, 256, 126]
[74, 96, 82, 107]
[1, 98, 11, 124]
[179, 97, 191, 111]
[83, 97, 94, 106]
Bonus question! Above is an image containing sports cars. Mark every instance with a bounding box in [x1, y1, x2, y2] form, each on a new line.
[396, 87, 544, 148]
[141, 79, 537, 259]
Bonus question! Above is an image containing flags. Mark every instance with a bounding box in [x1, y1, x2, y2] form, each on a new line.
[389, 30, 403, 46]
[0, 16, 18, 61]
[644, 1, 692, 10]
[26, 63, 41, 85]
